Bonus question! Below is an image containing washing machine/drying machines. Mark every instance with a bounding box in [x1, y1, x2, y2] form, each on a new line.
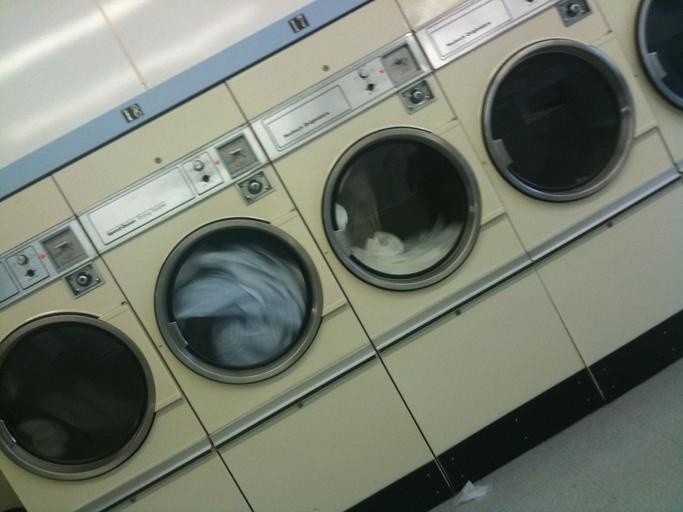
[0, 0, 683, 511]
